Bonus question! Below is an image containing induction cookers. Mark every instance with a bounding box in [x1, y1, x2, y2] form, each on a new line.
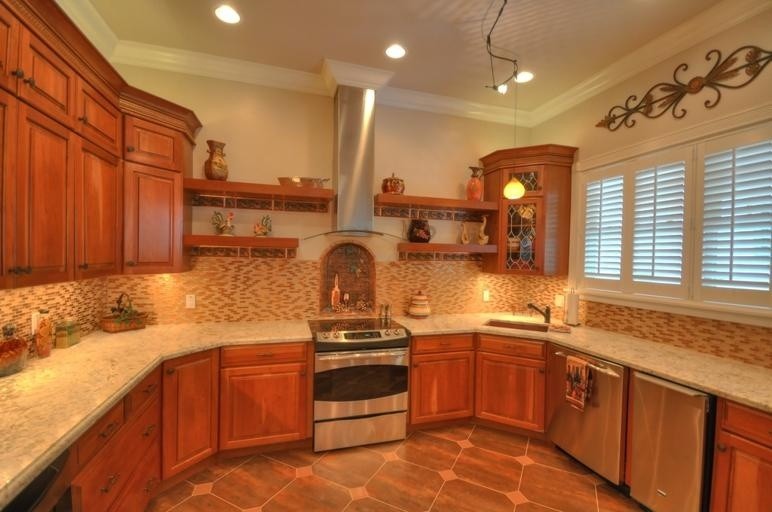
[307, 317, 412, 351]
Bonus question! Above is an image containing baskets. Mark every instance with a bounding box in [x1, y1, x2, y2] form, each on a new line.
[100, 292, 150, 333]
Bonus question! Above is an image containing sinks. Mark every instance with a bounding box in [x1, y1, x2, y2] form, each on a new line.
[485, 319, 549, 333]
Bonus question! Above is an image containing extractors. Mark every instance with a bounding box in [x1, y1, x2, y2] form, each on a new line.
[301, 84, 408, 244]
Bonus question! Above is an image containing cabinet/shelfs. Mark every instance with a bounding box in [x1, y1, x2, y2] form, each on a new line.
[75, 72, 123, 278]
[1, 363, 161, 512]
[475, 334, 545, 441]
[396, 242, 497, 261]
[630, 370, 709, 512]
[182, 178, 336, 213]
[710, 397, 772, 512]
[161, 346, 220, 493]
[0, 2, 75, 289]
[547, 342, 629, 487]
[374, 194, 498, 223]
[123, 115, 193, 274]
[183, 234, 299, 259]
[410, 334, 475, 432]
[220, 341, 314, 461]
[479, 144, 579, 275]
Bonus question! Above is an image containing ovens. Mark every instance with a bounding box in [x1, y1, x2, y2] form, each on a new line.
[314, 347, 410, 450]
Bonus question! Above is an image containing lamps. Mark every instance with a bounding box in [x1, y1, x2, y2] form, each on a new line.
[503, 173, 525, 199]
[214, 5, 241, 24]
[515, 71, 534, 83]
[493, 84, 507, 95]
[385, 44, 406, 59]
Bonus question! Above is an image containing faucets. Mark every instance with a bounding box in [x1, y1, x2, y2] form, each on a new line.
[528, 302, 550, 323]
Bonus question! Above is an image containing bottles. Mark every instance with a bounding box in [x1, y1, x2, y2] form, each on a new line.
[36, 309, 52, 358]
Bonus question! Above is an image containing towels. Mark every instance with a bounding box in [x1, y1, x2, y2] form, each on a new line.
[564, 354, 594, 414]
